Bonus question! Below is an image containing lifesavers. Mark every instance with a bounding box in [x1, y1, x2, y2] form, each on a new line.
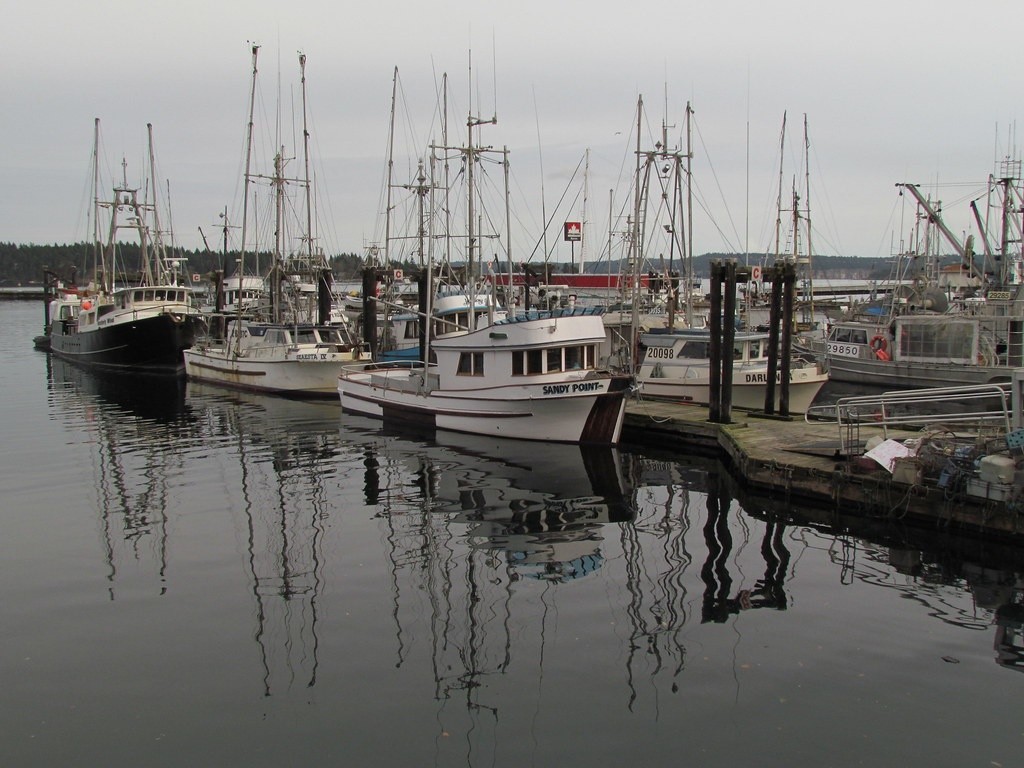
[876, 350, 891, 361]
[870, 335, 888, 352]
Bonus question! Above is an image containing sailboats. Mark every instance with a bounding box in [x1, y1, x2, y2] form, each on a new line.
[34, 26, 1024, 446]
